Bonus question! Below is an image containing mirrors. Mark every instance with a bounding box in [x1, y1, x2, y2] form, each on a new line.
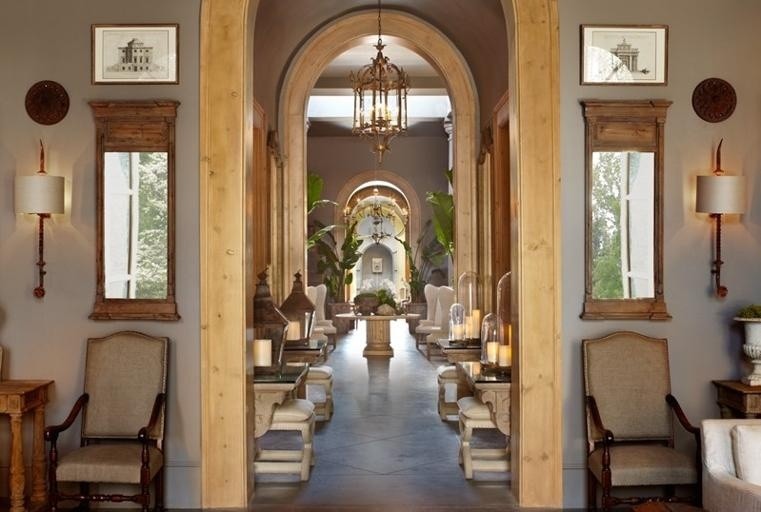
[579, 99, 672, 321]
[87, 100, 181, 320]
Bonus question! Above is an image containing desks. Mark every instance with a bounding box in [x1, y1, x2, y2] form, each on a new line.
[336, 314, 422, 358]
[0, 380, 55, 512]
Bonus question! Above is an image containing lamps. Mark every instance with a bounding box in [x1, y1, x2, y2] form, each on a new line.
[696, 138, 747, 298]
[347, 0, 412, 167]
[342, 187, 408, 245]
[13, 138, 64, 297]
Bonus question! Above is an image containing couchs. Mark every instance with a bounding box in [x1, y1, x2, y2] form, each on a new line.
[700, 418, 761, 512]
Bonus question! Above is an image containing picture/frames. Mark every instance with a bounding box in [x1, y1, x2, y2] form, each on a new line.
[579, 23, 668, 83]
[91, 23, 178, 85]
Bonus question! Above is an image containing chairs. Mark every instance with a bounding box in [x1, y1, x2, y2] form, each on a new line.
[254, 284, 338, 483]
[415, 285, 511, 482]
[44, 331, 169, 512]
[582, 331, 700, 512]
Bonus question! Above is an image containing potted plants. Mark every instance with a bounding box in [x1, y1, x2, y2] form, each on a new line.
[731, 305, 761, 386]
[313, 220, 364, 335]
[394, 219, 446, 334]
[353, 290, 402, 315]
